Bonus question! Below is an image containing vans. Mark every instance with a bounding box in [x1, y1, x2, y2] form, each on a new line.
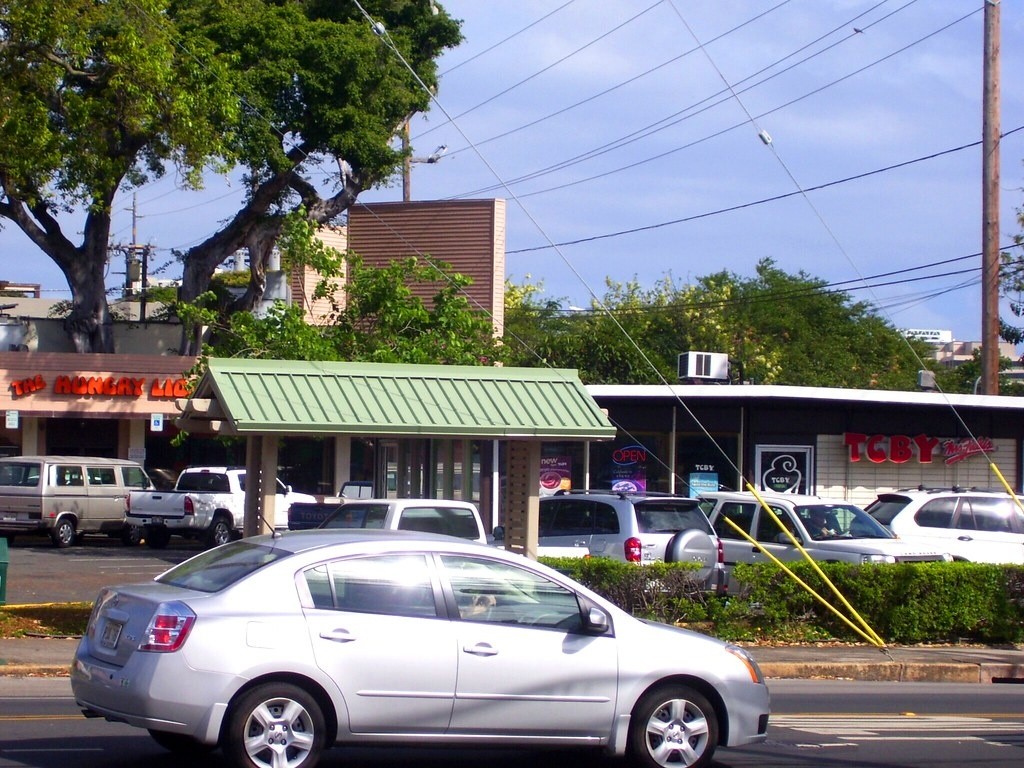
[0, 454, 160, 548]
[313, 498, 490, 585]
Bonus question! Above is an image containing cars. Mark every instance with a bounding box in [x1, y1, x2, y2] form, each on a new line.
[850, 488, 1024, 562]
[70, 527, 772, 768]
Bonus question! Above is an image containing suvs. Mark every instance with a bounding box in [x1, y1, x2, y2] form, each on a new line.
[710, 489, 945, 599]
[489, 487, 731, 612]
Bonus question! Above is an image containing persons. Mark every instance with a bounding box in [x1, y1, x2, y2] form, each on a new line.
[776, 509, 842, 544]
[459, 595, 495, 615]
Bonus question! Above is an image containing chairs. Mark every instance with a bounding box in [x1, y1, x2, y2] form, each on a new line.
[101, 472, 113, 484]
[68, 471, 82, 485]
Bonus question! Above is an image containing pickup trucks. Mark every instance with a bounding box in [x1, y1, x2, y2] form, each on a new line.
[122, 465, 316, 549]
[286, 480, 389, 532]
